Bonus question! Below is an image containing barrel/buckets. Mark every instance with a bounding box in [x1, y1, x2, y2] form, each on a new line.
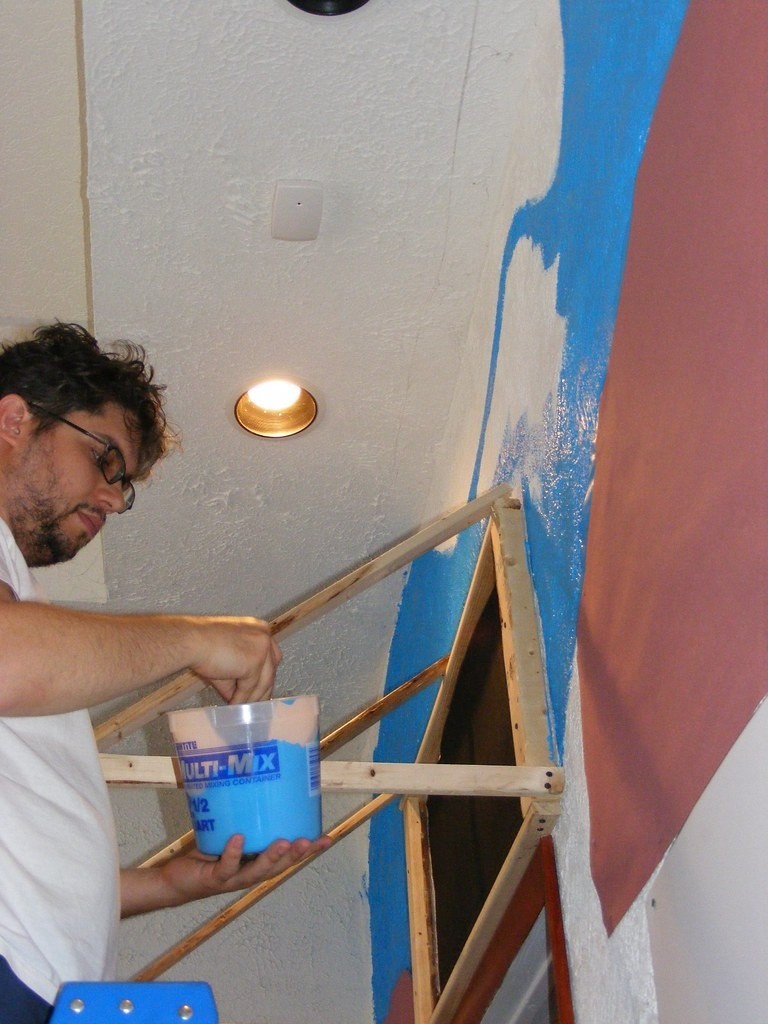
[163, 692, 321, 855]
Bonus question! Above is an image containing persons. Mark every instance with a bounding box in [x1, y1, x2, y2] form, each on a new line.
[0, 315, 333, 1024]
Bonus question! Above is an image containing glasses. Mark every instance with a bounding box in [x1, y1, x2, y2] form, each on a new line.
[27, 400, 135, 515]
